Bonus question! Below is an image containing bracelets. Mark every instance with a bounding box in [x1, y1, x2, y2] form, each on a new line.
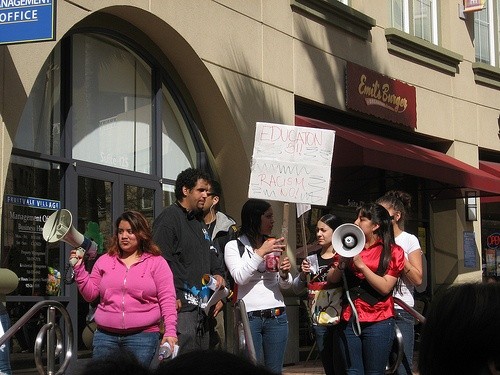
[280, 271, 288, 280]
[405, 265, 413, 275]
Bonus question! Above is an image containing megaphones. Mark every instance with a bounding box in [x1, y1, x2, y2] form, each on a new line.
[43, 209, 98, 267]
[331, 223, 366, 258]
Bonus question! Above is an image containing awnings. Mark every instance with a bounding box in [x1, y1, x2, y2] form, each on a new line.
[296, 116, 500, 203]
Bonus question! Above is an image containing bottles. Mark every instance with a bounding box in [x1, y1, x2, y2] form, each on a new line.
[158, 342, 171, 360]
[267, 235, 282, 273]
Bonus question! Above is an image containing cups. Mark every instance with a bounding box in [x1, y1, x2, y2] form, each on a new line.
[306, 254, 319, 274]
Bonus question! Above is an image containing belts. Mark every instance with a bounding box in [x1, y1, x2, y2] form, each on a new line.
[252, 307, 285, 318]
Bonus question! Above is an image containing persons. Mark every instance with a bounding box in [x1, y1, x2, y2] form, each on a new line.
[0, 169, 500, 375]
